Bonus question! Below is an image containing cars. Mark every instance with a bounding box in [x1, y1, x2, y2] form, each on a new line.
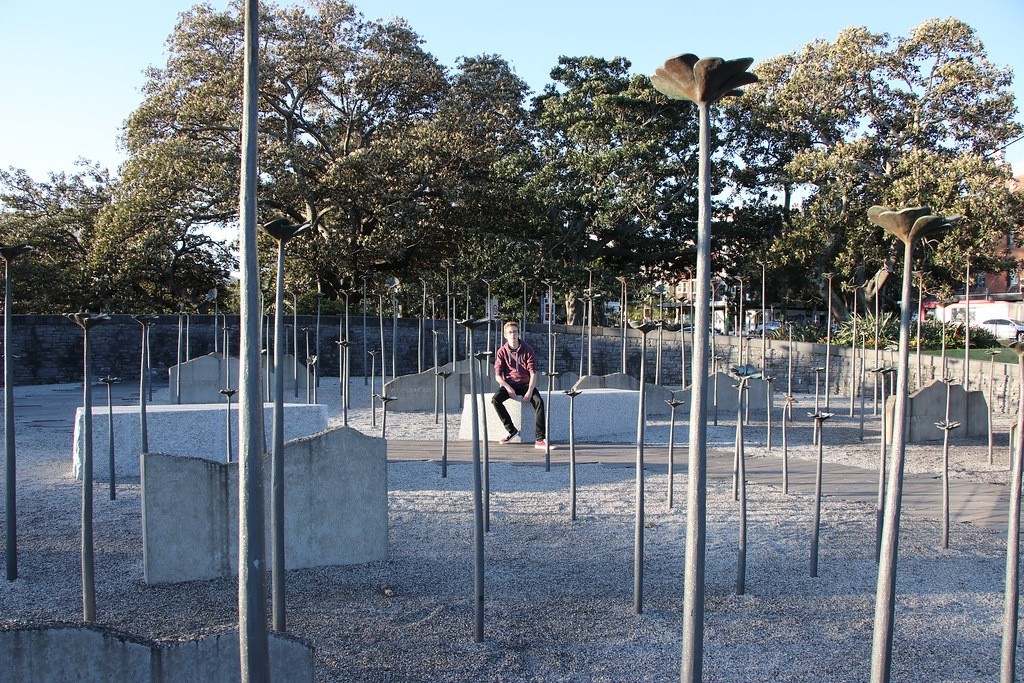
[969, 316, 1024, 346]
[675, 314, 789, 341]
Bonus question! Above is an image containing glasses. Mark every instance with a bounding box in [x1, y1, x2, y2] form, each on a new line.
[506, 330, 518, 335]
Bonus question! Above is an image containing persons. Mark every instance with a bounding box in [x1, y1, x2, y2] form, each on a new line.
[491, 322, 557, 451]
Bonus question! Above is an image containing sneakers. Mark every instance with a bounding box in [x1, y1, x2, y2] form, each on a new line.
[535, 439, 557, 450]
[499, 428, 519, 444]
